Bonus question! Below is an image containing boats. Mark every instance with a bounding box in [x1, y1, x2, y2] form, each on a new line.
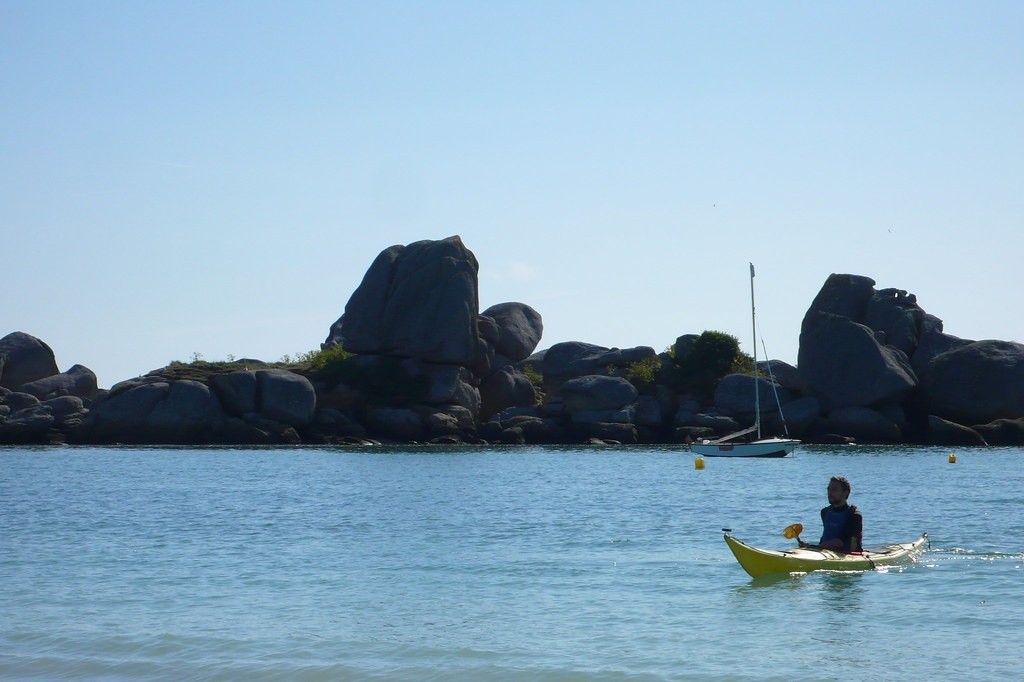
[720, 531, 929, 584]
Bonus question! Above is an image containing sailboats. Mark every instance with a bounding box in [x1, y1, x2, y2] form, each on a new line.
[689, 259, 803, 459]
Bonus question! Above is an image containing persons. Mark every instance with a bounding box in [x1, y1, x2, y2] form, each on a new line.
[802, 477, 863, 553]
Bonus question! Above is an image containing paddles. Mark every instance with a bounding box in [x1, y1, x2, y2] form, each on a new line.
[781, 521, 807, 547]
